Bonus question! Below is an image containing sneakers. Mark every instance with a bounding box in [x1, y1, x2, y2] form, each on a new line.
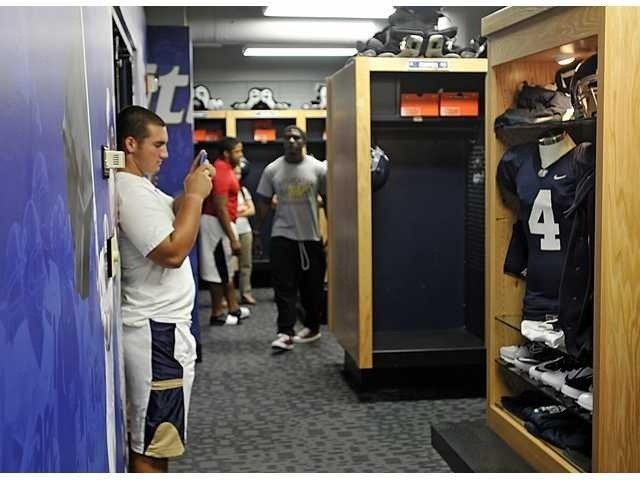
[291, 326, 322, 344]
[498, 343, 594, 413]
[227, 306, 251, 320]
[271, 332, 296, 351]
[241, 292, 257, 305]
[209, 313, 240, 327]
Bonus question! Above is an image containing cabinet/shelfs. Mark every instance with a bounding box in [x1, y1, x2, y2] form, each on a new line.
[479, 7, 640, 472]
[190, 104, 329, 146]
[321, 48, 489, 403]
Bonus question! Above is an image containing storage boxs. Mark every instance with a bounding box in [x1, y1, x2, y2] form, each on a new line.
[195, 128, 204, 142]
[252, 128, 277, 143]
[437, 89, 481, 119]
[398, 91, 441, 120]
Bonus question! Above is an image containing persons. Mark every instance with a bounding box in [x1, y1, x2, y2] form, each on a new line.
[199, 138, 250, 325]
[256, 126, 327, 350]
[114, 106, 216, 473]
[232, 165, 256, 304]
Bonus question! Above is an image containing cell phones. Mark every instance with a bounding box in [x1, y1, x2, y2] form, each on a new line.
[199, 152, 207, 166]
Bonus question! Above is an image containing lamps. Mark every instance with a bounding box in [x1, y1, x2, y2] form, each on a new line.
[258, 7, 402, 23]
[241, 42, 361, 60]
